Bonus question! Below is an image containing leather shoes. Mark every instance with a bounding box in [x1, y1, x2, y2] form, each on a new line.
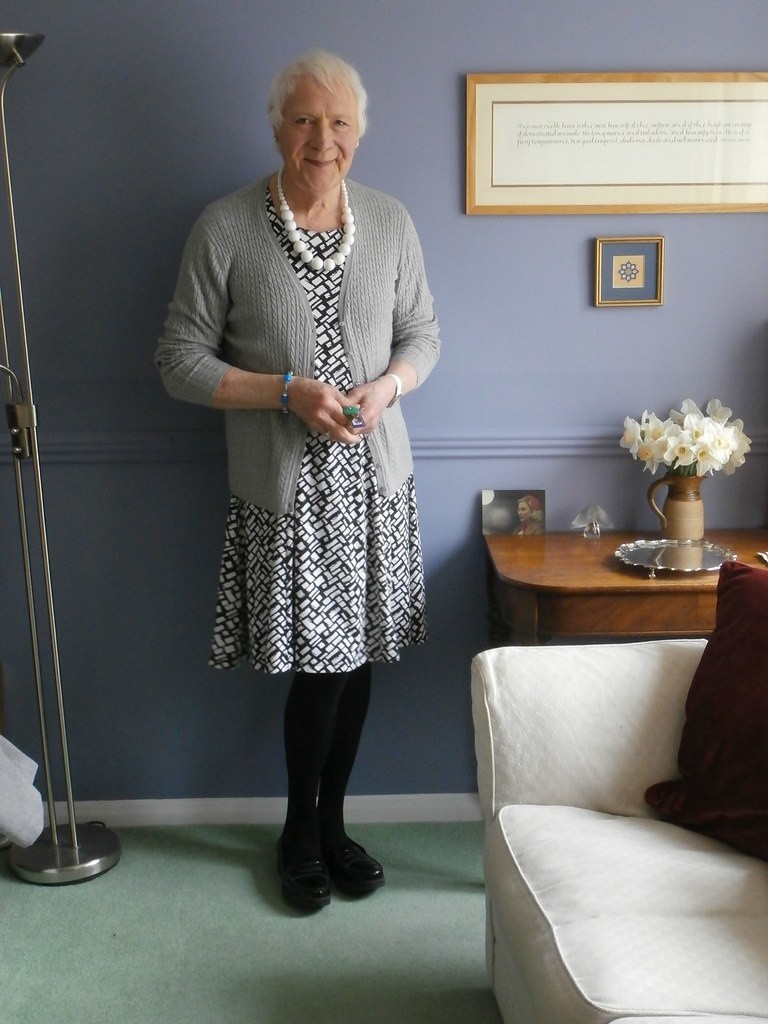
[325, 831, 386, 892]
[275, 838, 332, 909]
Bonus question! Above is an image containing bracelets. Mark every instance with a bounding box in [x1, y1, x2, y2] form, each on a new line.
[282, 371, 296, 415]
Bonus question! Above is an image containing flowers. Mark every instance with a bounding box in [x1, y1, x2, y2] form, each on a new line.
[620, 395, 752, 476]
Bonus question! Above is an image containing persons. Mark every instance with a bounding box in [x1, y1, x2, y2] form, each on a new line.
[512, 495, 543, 536]
[160, 48, 440, 908]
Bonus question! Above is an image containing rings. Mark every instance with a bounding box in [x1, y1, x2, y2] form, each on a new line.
[323, 430, 330, 441]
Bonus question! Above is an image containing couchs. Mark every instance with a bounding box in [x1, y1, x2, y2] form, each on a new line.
[471, 636, 767, 1023]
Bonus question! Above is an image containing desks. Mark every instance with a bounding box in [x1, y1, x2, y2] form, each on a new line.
[483, 526, 768, 645]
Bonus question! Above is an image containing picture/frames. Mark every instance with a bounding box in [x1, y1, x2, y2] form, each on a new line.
[466, 71, 768, 216]
[593, 235, 665, 307]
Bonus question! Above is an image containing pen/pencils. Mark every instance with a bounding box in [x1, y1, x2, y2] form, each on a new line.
[343, 408, 359, 416]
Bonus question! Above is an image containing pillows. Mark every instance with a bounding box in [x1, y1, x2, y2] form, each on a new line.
[644, 561, 767, 863]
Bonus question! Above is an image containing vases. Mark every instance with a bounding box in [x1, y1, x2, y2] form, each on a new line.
[646, 470, 709, 570]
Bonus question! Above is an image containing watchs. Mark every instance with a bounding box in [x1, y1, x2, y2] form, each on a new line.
[386, 372, 402, 408]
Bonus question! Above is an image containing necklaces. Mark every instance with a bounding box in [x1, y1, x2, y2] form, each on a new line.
[278, 170, 356, 271]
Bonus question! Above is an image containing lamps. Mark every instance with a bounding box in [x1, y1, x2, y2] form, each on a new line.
[0, 31, 123, 886]
